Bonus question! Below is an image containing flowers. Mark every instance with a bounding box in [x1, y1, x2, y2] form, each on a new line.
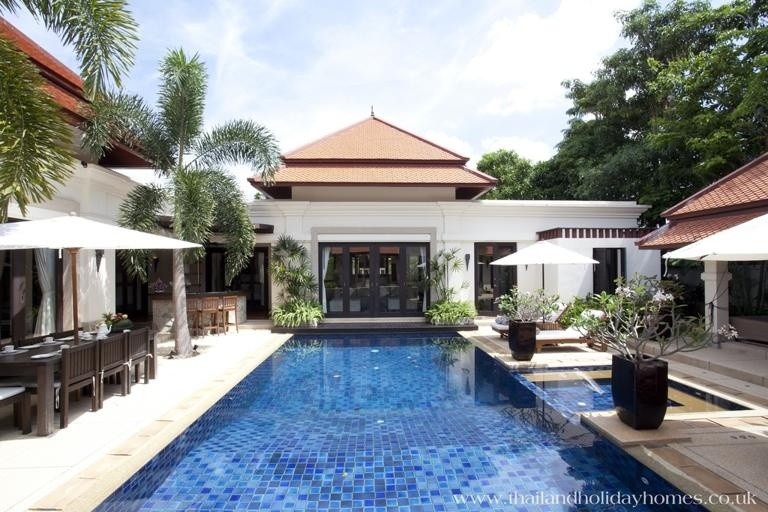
[152, 278, 168, 291]
[493, 286, 560, 325]
[568, 272, 739, 368]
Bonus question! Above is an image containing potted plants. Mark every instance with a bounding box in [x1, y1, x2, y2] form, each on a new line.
[267, 235, 325, 329]
[416, 246, 478, 325]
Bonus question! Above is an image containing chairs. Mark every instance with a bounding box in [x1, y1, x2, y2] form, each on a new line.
[492, 302, 568, 338]
[0, 322, 158, 435]
[535, 310, 608, 352]
[187, 295, 238, 339]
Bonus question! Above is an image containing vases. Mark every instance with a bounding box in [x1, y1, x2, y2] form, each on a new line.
[509, 321, 536, 361]
[155, 290, 164, 293]
[612, 354, 668, 430]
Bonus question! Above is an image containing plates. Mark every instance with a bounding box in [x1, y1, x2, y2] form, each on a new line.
[0, 350, 18, 353]
[30, 354, 54, 359]
[42, 341, 56, 344]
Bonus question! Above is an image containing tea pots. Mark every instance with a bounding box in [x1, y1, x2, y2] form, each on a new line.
[95, 321, 113, 335]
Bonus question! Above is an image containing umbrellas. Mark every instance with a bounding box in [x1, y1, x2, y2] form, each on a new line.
[487, 237, 601, 321]
[0, 212, 204, 345]
[662, 212, 767, 262]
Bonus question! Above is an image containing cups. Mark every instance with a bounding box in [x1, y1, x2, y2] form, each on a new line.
[1, 345, 15, 351]
[43, 337, 53, 342]
[78, 331, 91, 339]
